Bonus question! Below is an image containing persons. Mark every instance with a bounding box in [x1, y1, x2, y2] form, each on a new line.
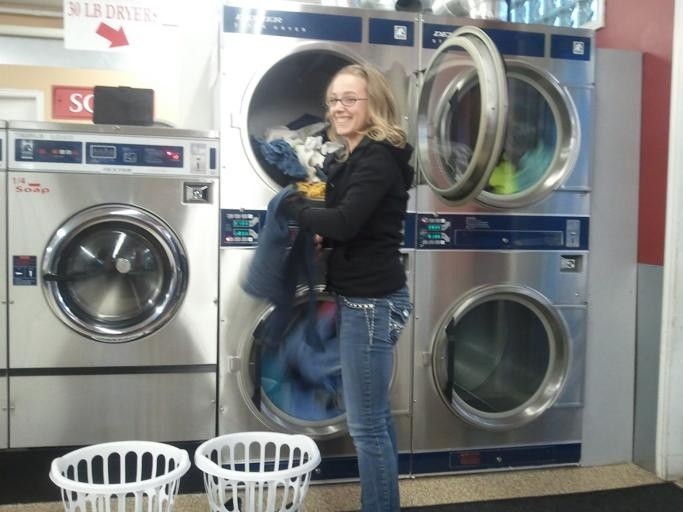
[279, 65, 415, 512]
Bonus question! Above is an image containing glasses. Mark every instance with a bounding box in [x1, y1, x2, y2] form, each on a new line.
[324, 95, 368, 107]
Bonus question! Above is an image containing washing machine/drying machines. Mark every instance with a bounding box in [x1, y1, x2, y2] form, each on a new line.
[216, 6, 593, 491]
[6, 122, 220, 501]
